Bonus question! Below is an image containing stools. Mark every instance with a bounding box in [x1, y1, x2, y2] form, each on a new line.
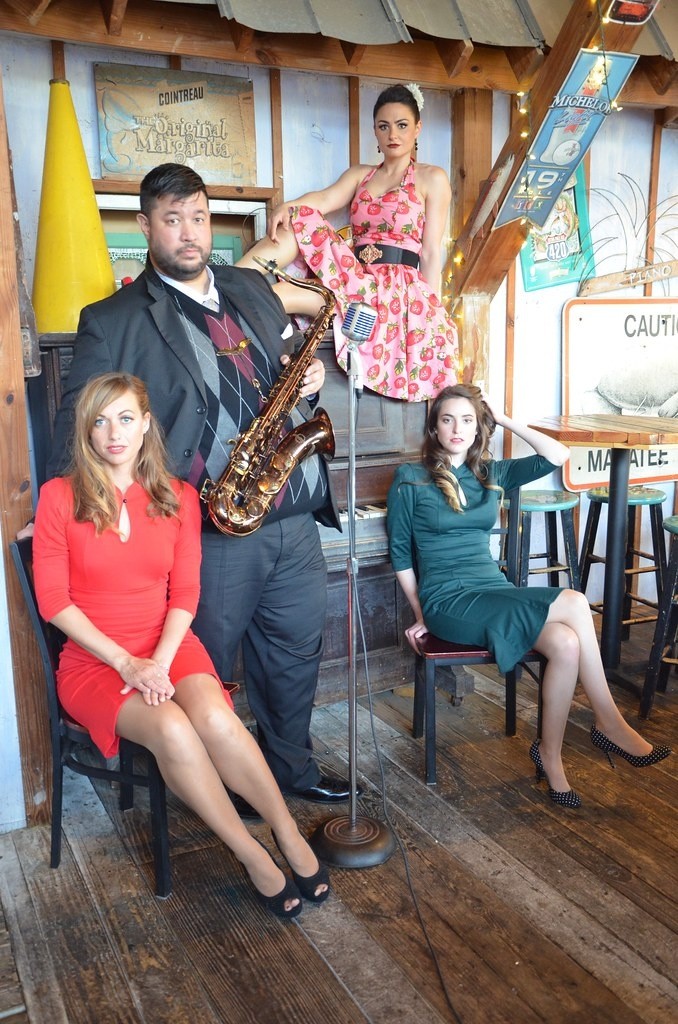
[498, 485, 678, 721]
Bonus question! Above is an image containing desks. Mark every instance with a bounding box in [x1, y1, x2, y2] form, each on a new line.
[528, 414, 678, 699]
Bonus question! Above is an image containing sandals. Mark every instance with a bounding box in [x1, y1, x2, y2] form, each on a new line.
[269, 825, 331, 903]
[236, 835, 303, 917]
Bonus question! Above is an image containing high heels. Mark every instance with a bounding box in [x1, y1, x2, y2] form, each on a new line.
[528, 738, 583, 809]
[589, 722, 672, 770]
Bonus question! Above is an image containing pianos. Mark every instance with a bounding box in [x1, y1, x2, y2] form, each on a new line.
[34, 327, 477, 709]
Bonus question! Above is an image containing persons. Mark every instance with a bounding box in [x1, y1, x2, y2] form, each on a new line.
[120, 82, 458, 403]
[17, 161, 358, 799]
[31, 372, 333, 918]
[386, 383, 673, 809]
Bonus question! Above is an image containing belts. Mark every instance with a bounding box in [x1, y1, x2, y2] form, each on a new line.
[354, 244, 419, 269]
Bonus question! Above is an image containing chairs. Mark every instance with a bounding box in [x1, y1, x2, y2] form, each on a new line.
[411, 531, 549, 786]
[10, 536, 173, 900]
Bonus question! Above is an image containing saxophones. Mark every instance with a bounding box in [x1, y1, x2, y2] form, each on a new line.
[198, 256, 336, 540]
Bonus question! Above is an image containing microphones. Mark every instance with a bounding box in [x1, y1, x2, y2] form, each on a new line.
[339, 300, 378, 344]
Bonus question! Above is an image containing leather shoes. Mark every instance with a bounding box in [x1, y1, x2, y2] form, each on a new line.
[285, 775, 362, 805]
[225, 787, 264, 820]
